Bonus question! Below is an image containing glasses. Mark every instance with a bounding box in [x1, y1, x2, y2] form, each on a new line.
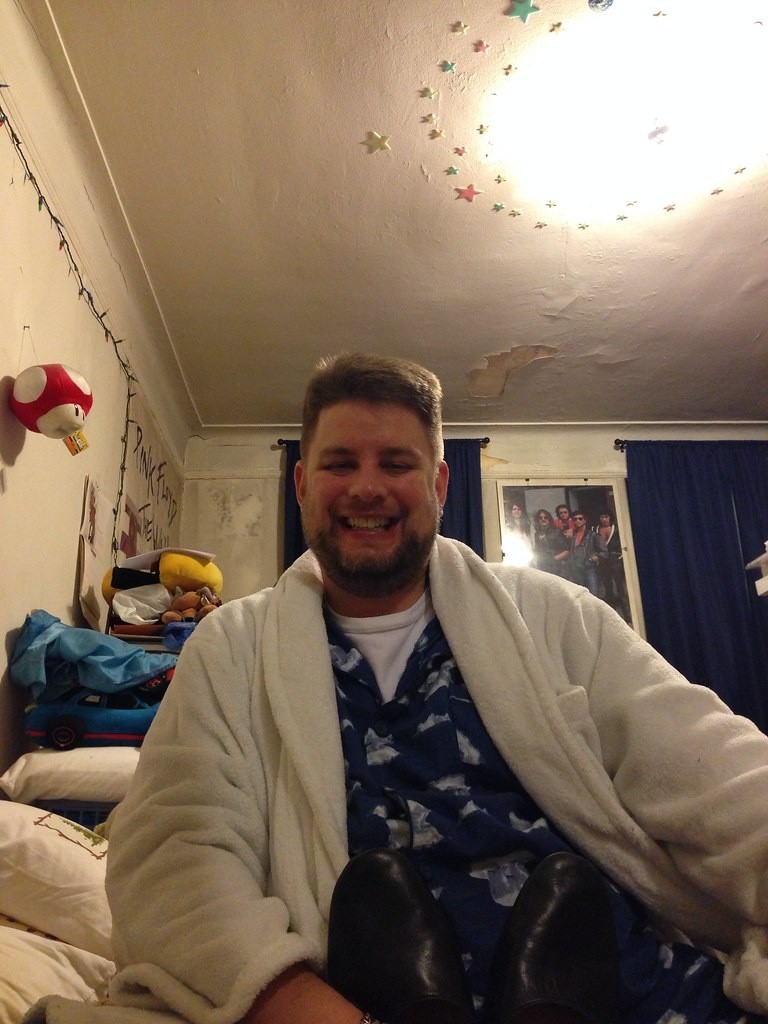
[572, 517, 584, 521]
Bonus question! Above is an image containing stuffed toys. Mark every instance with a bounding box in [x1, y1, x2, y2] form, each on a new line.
[101, 553, 222, 626]
[9, 364, 93, 439]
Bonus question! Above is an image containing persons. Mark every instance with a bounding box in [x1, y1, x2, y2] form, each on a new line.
[505, 500, 631, 626]
[102, 351, 767, 1024]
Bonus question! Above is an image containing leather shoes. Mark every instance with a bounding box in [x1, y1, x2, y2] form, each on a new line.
[326, 846, 475, 1024]
[499, 850, 625, 1024]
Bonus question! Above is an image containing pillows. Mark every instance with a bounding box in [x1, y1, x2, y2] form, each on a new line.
[0, 746, 139, 807]
[0, 924, 116, 1024]
[0, 800, 114, 962]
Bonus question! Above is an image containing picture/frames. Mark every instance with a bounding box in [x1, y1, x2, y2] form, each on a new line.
[496, 477, 641, 639]
